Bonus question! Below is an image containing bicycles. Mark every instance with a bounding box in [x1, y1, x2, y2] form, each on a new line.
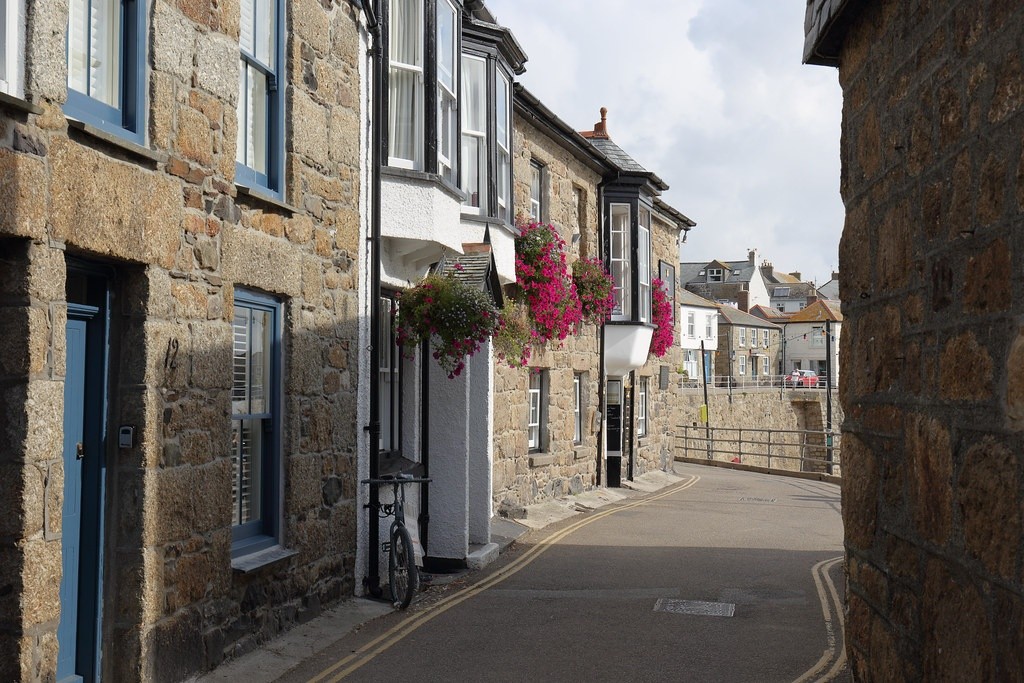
[361, 475, 434, 609]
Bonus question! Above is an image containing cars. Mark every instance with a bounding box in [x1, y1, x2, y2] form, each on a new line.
[783, 369, 820, 388]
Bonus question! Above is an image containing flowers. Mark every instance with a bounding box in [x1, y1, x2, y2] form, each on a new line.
[392, 218, 619, 379]
[649, 278, 675, 357]
